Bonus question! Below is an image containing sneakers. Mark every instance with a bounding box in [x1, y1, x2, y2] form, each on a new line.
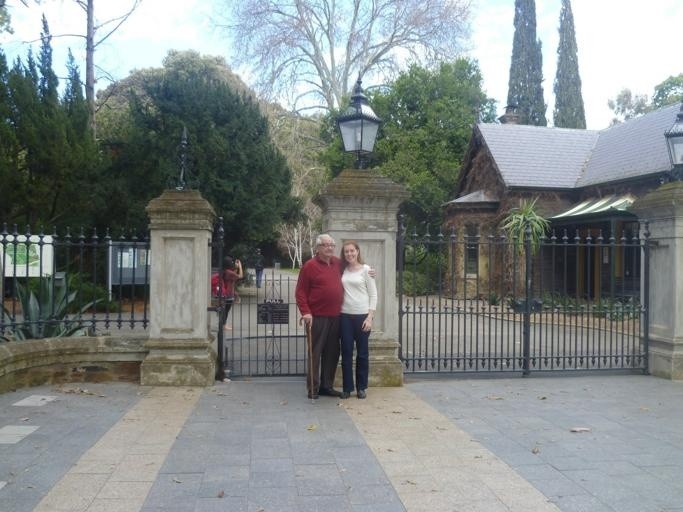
[319, 388, 343, 397]
[308, 390, 319, 400]
[357, 390, 367, 400]
[340, 392, 351, 399]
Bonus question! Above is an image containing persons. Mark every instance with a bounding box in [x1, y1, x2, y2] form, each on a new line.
[220, 256, 243, 330]
[295, 234, 378, 399]
[253, 249, 265, 288]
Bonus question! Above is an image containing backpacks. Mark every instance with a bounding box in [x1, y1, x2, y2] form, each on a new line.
[212, 272, 227, 300]
